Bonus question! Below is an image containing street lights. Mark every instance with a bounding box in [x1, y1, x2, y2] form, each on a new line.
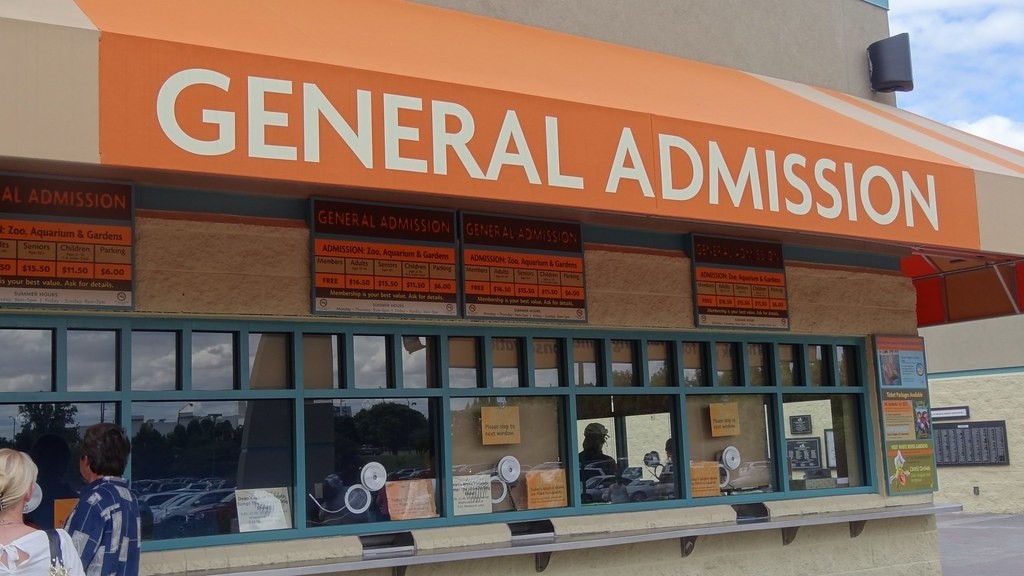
[177, 403, 193, 426]
[207, 413, 224, 475]
[7, 415, 15, 450]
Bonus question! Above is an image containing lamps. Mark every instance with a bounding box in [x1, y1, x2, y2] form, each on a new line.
[866, 33, 914, 92]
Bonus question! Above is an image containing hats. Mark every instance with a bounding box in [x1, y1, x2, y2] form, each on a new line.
[584, 423, 608, 436]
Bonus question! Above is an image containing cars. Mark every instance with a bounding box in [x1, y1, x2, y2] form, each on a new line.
[183, 491, 238, 533]
[130, 476, 235, 520]
[385, 461, 564, 481]
[152, 487, 237, 538]
[577, 455, 676, 503]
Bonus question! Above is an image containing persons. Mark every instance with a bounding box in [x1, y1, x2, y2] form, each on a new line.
[660, 438, 675, 472]
[579, 423, 616, 481]
[602, 481, 630, 504]
[0, 448, 87, 576]
[62, 421, 141, 576]
